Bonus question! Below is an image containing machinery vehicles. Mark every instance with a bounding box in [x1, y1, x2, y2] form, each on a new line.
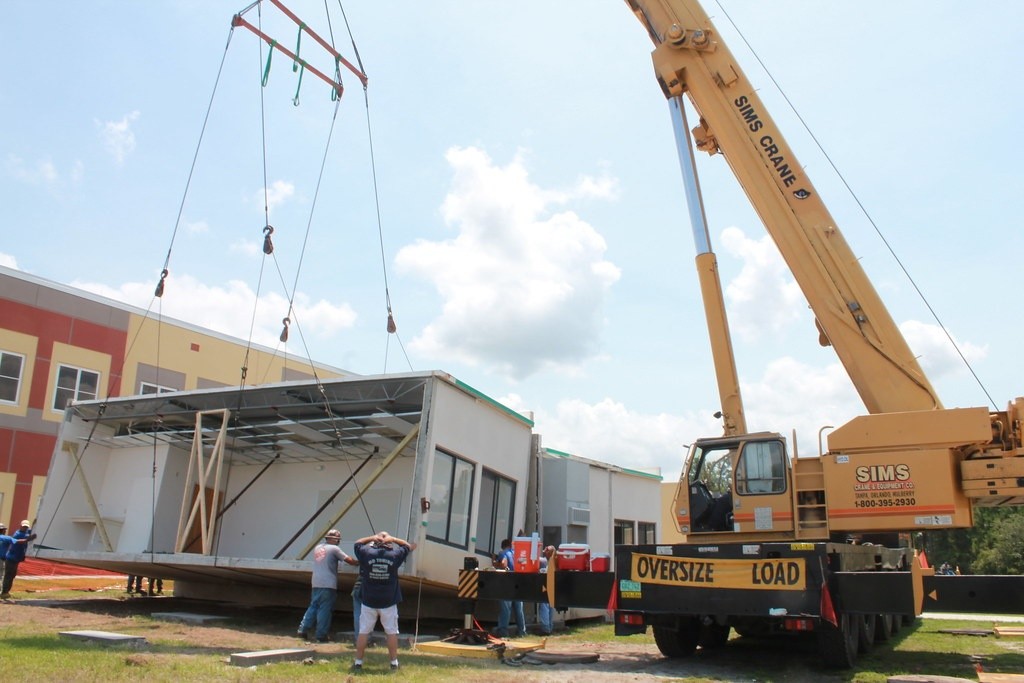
[612, 1, 1024, 667]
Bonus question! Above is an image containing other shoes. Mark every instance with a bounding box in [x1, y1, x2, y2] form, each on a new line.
[390, 659, 398, 670]
[368, 641, 377, 648]
[354, 658, 362, 669]
[312, 635, 334, 643]
[0, 597, 5, 601]
[538, 632, 550, 636]
[157, 591, 164, 596]
[136, 591, 146, 595]
[149, 592, 158, 596]
[127, 589, 136, 593]
[3, 598, 16, 604]
[296, 631, 307, 640]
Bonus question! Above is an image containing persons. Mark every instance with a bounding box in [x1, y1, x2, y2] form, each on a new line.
[0, 519, 36, 597]
[127, 574, 145, 594]
[536, 545, 555, 635]
[695, 485, 732, 532]
[148, 577, 164, 596]
[491, 539, 528, 638]
[349, 531, 417, 670]
[297, 529, 359, 642]
[0, 523, 37, 591]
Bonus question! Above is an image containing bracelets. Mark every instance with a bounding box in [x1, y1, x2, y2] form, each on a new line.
[493, 561, 497, 563]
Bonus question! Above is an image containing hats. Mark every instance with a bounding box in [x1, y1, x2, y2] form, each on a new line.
[324, 530, 342, 539]
[20, 520, 29, 527]
[0, 523, 7, 529]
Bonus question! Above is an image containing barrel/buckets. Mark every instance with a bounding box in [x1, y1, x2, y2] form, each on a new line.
[511, 533, 543, 573]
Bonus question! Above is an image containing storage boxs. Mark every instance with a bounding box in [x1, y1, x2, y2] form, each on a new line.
[556, 543, 591, 571]
[591, 552, 611, 572]
